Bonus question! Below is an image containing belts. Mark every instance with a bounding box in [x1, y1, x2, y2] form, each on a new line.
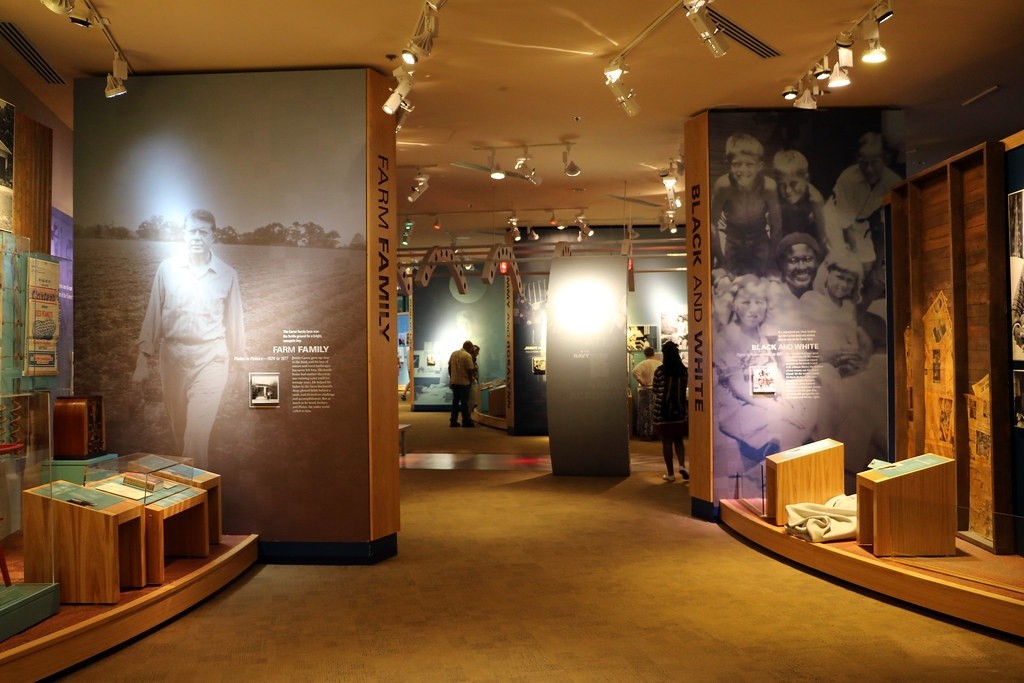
[165, 337, 203, 346]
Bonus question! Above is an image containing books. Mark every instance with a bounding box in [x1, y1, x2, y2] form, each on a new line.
[124, 472, 165, 492]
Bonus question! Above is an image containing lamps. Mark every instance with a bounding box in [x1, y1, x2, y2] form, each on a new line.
[507, 208, 596, 241]
[381, 0, 447, 116]
[683, 0, 730, 58]
[488, 146, 581, 186]
[408, 241, 472, 271]
[112, 50, 127, 81]
[658, 155, 685, 233]
[40, 0, 75, 15]
[602, 57, 640, 118]
[780, 0, 895, 108]
[69, 8, 95, 28]
[407, 174, 429, 203]
[103, 73, 126, 98]
[627, 220, 640, 239]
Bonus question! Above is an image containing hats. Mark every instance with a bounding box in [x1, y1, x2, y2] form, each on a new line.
[866, 298, 886, 319]
[777, 232, 821, 260]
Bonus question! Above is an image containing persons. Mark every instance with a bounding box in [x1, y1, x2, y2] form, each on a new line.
[447, 341, 481, 428]
[708, 133, 900, 496]
[1013, 263, 1024, 352]
[400, 348, 411, 401]
[131, 210, 248, 473]
[632, 341, 689, 480]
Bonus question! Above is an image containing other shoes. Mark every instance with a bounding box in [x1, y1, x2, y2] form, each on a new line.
[663, 474, 675, 481]
[678, 466, 689, 479]
[462, 422, 474, 427]
[450, 422, 459, 427]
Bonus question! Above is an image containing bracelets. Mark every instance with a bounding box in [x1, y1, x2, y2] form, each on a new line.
[1013, 323, 1021, 335]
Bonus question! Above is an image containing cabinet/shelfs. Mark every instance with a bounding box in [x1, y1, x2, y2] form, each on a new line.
[41, 454, 118, 486]
